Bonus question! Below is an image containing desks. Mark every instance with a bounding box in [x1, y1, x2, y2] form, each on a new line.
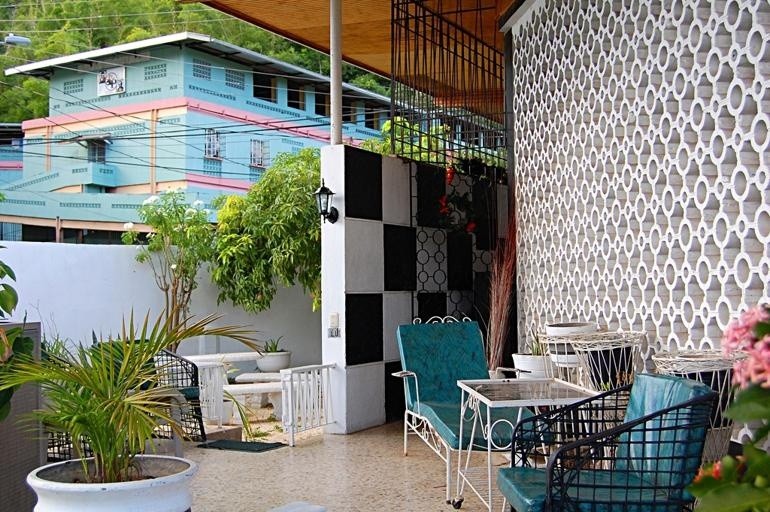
[452, 379, 610, 512]
[188, 362, 223, 428]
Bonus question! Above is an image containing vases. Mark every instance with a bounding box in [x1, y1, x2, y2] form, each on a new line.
[547, 323, 733, 462]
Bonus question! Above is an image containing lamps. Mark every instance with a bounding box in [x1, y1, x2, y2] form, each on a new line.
[313, 178, 339, 224]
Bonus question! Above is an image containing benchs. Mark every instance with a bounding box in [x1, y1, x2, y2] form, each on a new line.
[224, 382, 282, 421]
[235, 372, 320, 409]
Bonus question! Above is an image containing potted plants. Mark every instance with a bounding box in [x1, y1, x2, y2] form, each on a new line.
[511, 329, 557, 379]
[0, 306, 265, 512]
[256, 335, 292, 373]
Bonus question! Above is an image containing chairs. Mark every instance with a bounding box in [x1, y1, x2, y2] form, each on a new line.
[93, 340, 207, 443]
[391, 316, 555, 504]
[497, 372, 719, 512]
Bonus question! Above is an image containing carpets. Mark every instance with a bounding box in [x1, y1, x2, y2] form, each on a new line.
[197, 439, 287, 451]
